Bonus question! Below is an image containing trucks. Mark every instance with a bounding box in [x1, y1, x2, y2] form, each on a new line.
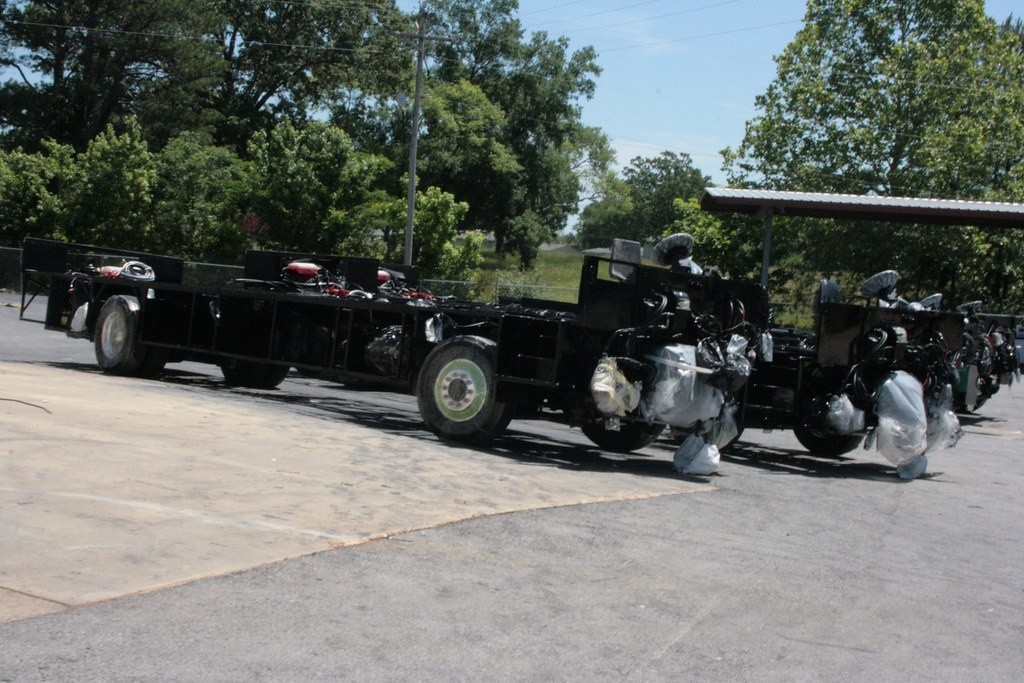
[19, 233, 1017, 476]
